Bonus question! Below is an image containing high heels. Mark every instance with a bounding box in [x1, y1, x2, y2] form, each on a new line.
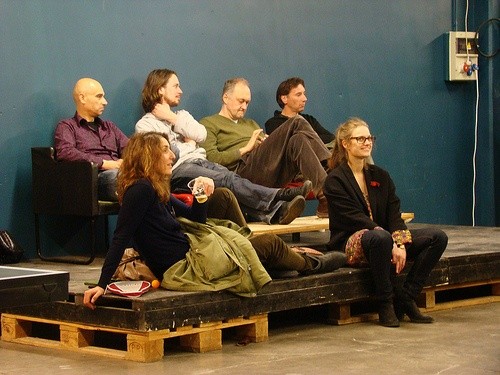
[378, 298, 400, 327]
[396, 293, 434, 323]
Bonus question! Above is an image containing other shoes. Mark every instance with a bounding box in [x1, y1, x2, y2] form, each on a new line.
[316, 199, 329, 218]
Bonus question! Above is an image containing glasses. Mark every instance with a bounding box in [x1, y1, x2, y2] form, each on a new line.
[350, 135, 376, 144]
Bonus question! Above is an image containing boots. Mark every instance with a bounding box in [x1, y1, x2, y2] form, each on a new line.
[297, 252, 348, 273]
[278, 195, 306, 224]
[277, 179, 312, 201]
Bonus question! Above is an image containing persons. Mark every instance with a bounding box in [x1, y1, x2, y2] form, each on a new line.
[321, 119, 448, 327]
[55, 78, 131, 201]
[198, 77, 333, 218]
[265, 77, 337, 145]
[83, 132, 346, 310]
[135, 69, 312, 225]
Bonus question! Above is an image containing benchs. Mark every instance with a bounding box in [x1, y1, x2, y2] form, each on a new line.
[170, 181, 317, 241]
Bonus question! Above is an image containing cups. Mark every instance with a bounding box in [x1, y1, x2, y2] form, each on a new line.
[187, 179, 208, 204]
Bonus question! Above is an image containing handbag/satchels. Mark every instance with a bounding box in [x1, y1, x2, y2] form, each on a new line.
[0, 229, 25, 264]
[112, 247, 161, 286]
[290, 245, 324, 257]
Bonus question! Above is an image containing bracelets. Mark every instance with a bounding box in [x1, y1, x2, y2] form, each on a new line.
[397, 245, 404, 249]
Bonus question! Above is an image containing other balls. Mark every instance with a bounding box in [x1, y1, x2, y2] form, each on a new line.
[152, 280, 160, 288]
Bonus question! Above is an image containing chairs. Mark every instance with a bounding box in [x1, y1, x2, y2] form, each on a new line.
[31, 145, 119, 267]
